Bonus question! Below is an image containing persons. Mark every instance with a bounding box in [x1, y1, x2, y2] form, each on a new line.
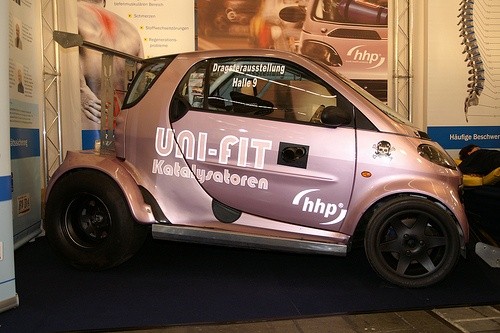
[76, 0, 145, 131]
[458, 143, 481, 160]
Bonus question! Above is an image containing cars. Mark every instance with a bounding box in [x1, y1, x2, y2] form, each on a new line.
[276, 0, 388, 104]
[40, 30, 470, 289]
